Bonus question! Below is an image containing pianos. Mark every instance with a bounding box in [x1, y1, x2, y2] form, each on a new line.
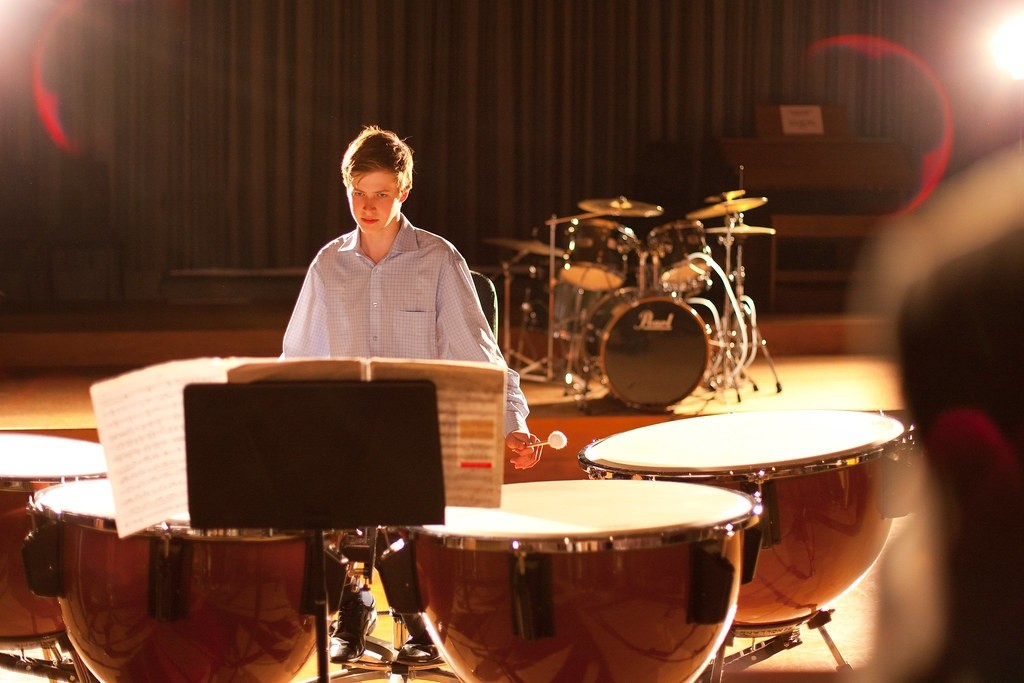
[709, 102, 917, 310]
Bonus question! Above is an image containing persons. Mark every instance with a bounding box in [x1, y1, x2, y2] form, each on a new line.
[281, 128, 544, 665]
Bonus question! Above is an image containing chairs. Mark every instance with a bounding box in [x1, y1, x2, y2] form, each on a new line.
[304, 268, 501, 683]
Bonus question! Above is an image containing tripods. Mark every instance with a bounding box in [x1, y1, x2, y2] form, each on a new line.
[500, 223, 584, 396]
[706, 213, 783, 405]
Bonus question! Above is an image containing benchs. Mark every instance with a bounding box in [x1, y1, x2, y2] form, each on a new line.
[748, 212, 874, 312]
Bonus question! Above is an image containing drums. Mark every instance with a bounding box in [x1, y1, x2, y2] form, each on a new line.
[581, 288, 709, 410]
[25, 477, 353, 683]
[558, 219, 637, 290]
[576, 409, 916, 638]
[410, 479, 765, 683]
[649, 220, 711, 284]
[0, 431, 109, 650]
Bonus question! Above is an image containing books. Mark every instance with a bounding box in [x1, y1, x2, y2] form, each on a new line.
[227, 357, 505, 508]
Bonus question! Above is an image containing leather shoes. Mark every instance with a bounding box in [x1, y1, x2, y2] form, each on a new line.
[329, 598, 379, 664]
[396, 631, 442, 665]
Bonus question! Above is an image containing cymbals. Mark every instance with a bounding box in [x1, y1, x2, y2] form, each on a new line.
[576, 198, 664, 217]
[705, 188, 745, 203]
[705, 224, 777, 235]
[686, 197, 768, 219]
[484, 238, 566, 256]
